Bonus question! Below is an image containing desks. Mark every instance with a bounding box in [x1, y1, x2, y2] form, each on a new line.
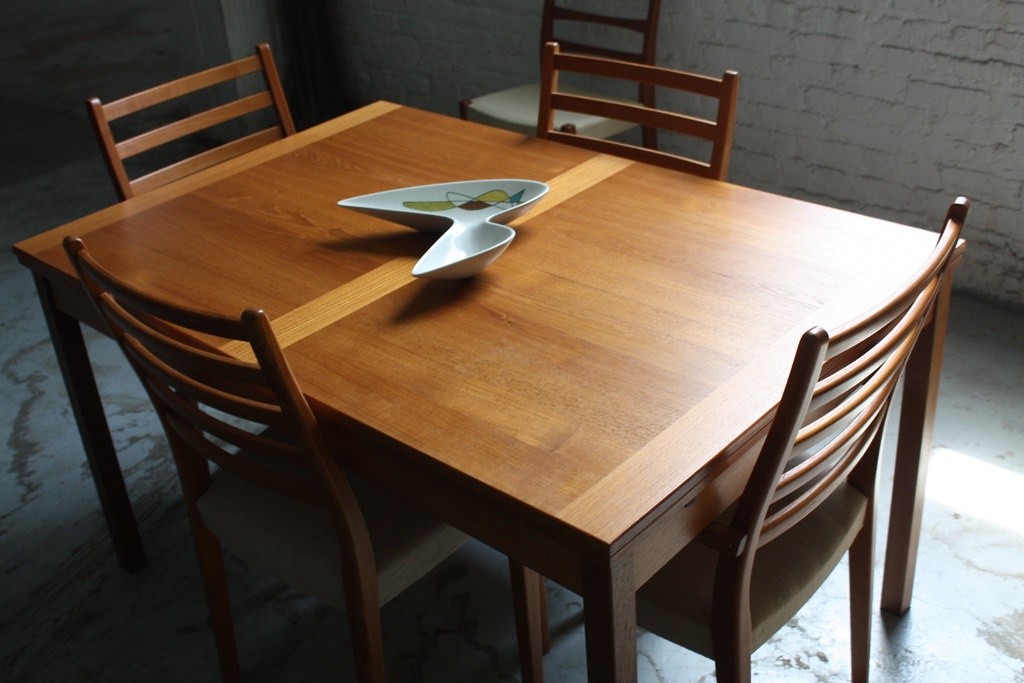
[8, 98, 969, 683]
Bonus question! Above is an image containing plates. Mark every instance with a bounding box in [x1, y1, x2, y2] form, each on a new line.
[337, 178, 549, 278]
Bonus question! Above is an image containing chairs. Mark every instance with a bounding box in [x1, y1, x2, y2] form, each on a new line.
[511, 195, 968, 683]
[61, 230, 544, 683]
[537, 41, 741, 179]
[86, 44, 296, 203]
[460, 0, 660, 152]
